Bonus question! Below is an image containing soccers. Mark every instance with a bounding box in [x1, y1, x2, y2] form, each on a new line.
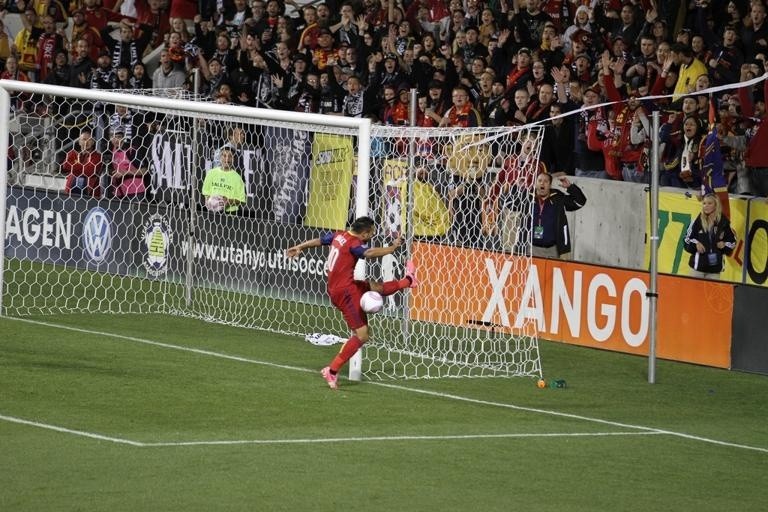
[207, 196, 225, 211]
[360, 290, 384, 314]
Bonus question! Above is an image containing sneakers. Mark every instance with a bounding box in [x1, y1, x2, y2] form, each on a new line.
[321, 366, 340, 391]
[406, 259, 419, 288]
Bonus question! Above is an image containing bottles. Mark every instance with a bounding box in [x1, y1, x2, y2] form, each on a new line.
[536, 377, 568, 388]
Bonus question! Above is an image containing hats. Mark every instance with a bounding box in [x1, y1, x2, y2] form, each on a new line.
[575, 51, 590, 62]
[465, 25, 481, 35]
[220, 143, 236, 154]
[383, 53, 396, 60]
[519, 47, 531, 56]
[583, 85, 601, 94]
[629, 89, 640, 97]
[111, 125, 124, 134]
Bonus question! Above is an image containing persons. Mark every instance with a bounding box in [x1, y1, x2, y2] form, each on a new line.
[283, 215, 420, 390]
[0, 0, 768, 283]
